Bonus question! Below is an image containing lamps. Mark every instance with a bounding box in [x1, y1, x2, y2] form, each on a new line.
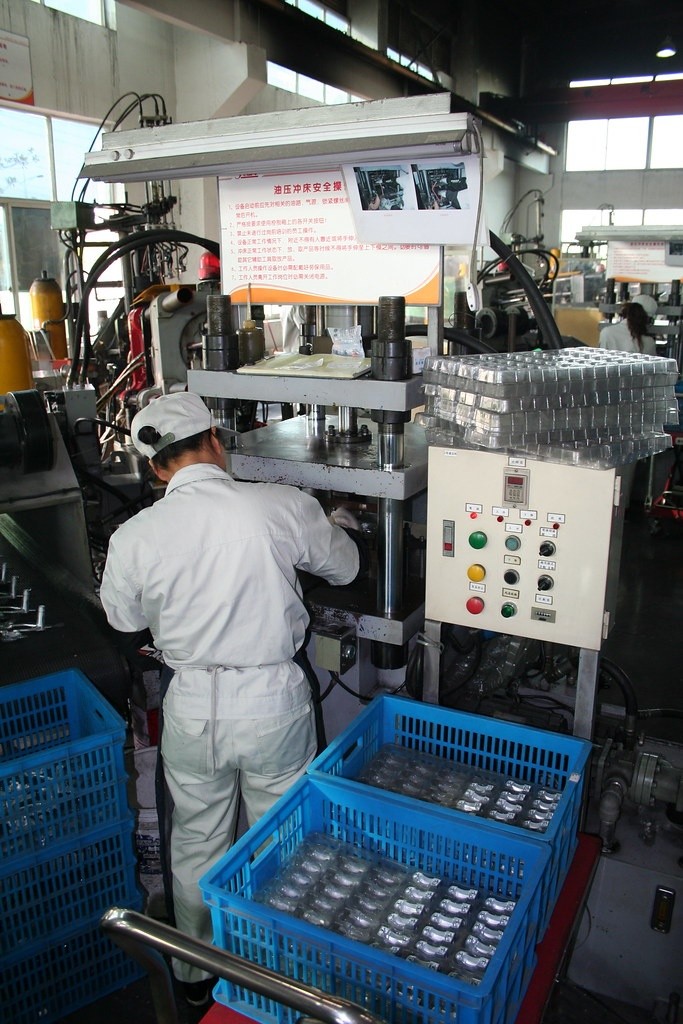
[79, 112, 484, 184]
[655, 36, 678, 59]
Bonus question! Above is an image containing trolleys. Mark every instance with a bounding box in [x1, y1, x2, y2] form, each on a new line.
[96, 830, 613, 1024]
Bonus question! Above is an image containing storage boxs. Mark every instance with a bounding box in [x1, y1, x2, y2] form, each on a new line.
[198, 692, 592, 1024]
[0, 669, 150, 1024]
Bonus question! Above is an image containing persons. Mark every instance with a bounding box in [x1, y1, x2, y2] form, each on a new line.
[101, 390, 364, 1006]
[279, 305, 305, 417]
[598, 293, 658, 562]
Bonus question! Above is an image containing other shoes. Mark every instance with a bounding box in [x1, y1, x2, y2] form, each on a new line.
[185, 983, 209, 1007]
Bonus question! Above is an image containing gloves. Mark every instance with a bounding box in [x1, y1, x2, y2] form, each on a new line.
[331, 508, 361, 530]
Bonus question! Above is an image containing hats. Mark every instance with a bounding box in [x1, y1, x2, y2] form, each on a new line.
[632, 295, 658, 317]
[130, 391, 240, 460]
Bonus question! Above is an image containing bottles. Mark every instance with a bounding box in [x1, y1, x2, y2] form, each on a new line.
[236, 319, 265, 367]
